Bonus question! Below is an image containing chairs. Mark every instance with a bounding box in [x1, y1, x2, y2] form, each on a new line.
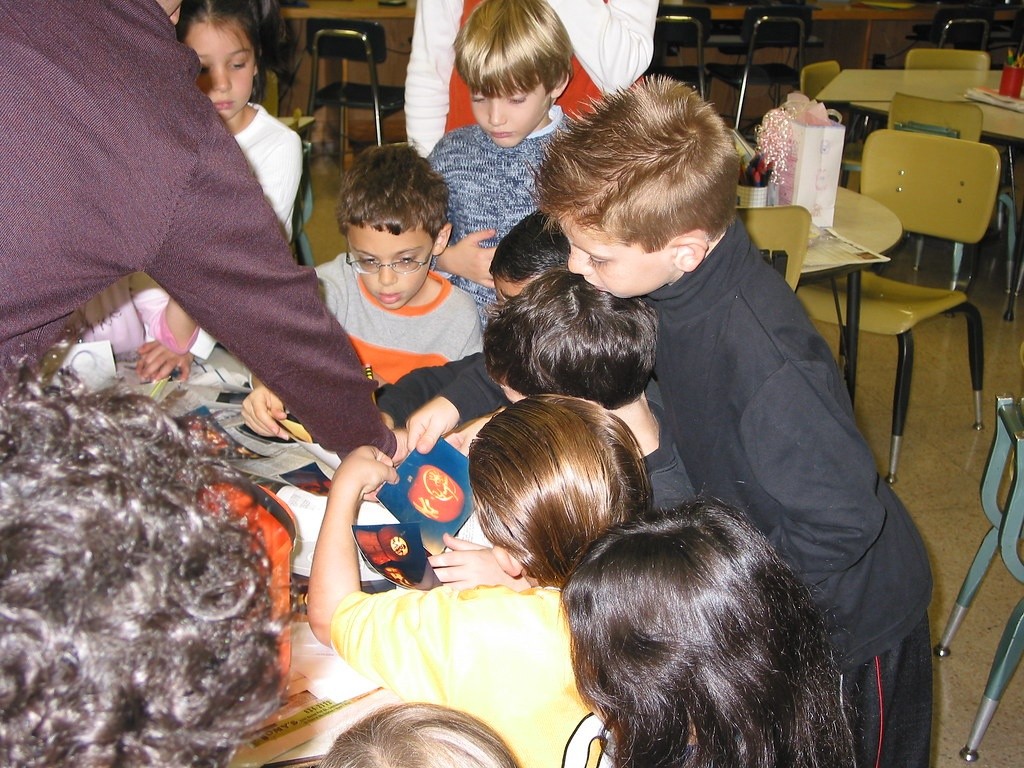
[296, 0, 1024, 762]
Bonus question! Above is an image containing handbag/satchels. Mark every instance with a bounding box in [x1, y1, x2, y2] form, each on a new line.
[772, 108, 846, 228]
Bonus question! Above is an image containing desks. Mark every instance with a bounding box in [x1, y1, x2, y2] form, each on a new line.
[813, 68, 1024, 303]
[250, 0, 420, 162]
[660, 4, 1021, 69]
[735, 186, 903, 414]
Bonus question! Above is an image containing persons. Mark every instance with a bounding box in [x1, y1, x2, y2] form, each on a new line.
[0, 368, 288, 768]
[82, 0, 934, 768]
[0, 0, 408, 460]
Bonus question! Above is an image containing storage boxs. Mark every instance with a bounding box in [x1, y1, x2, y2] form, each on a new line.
[756, 111, 846, 228]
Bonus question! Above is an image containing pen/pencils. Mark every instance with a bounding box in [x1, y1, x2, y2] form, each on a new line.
[739, 153, 775, 187]
[365, 363, 377, 404]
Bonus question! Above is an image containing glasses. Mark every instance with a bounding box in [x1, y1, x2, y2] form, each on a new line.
[345, 232, 434, 274]
